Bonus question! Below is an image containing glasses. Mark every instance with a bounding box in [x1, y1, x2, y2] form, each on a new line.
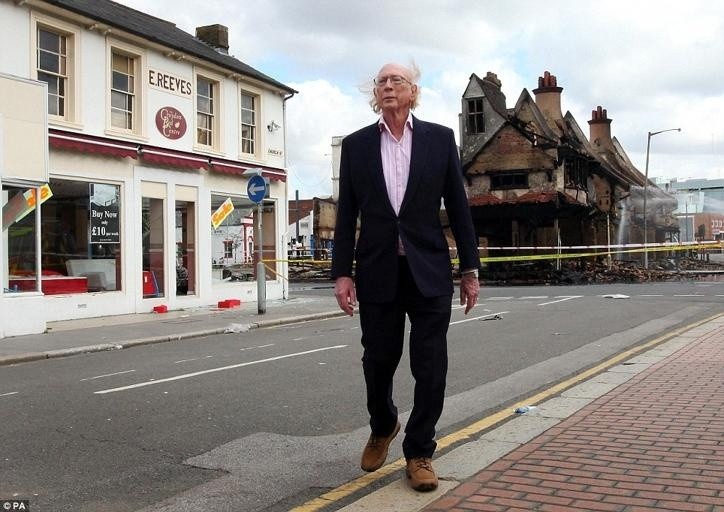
[373, 74, 412, 85]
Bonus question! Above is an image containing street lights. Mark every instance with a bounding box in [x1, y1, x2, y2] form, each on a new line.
[606, 192, 633, 270]
[641, 128, 681, 270]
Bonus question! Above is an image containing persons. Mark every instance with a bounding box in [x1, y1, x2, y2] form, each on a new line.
[331, 62, 480, 490]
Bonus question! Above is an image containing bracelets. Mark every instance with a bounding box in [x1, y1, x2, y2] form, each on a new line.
[460, 269, 477, 275]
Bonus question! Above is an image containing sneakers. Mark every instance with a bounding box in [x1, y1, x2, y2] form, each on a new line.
[361, 419, 400, 472]
[406, 457, 438, 492]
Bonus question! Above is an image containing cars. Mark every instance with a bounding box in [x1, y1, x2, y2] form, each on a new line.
[143, 249, 163, 290]
